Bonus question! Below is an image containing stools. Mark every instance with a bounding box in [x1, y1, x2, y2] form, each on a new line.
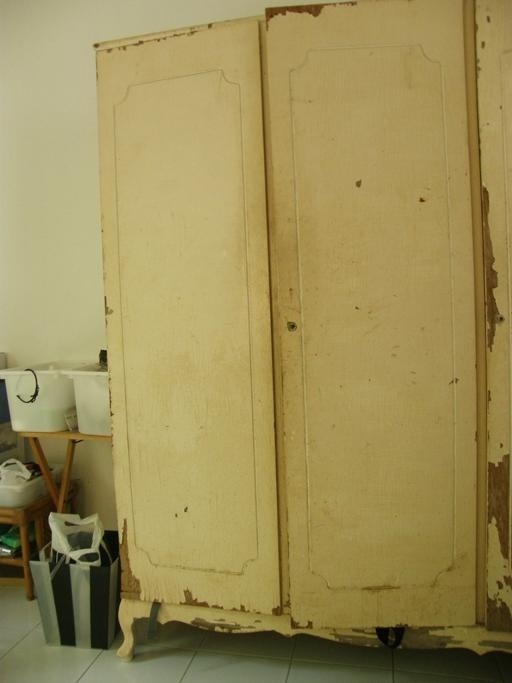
[0, 472, 79, 602]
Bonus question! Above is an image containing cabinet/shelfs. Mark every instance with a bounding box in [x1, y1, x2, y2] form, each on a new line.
[90, 0, 512, 664]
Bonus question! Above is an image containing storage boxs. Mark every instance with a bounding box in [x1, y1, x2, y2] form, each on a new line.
[65, 363, 115, 440]
[2, 367, 72, 435]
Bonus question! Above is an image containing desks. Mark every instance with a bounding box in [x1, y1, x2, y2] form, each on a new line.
[10, 424, 114, 541]
